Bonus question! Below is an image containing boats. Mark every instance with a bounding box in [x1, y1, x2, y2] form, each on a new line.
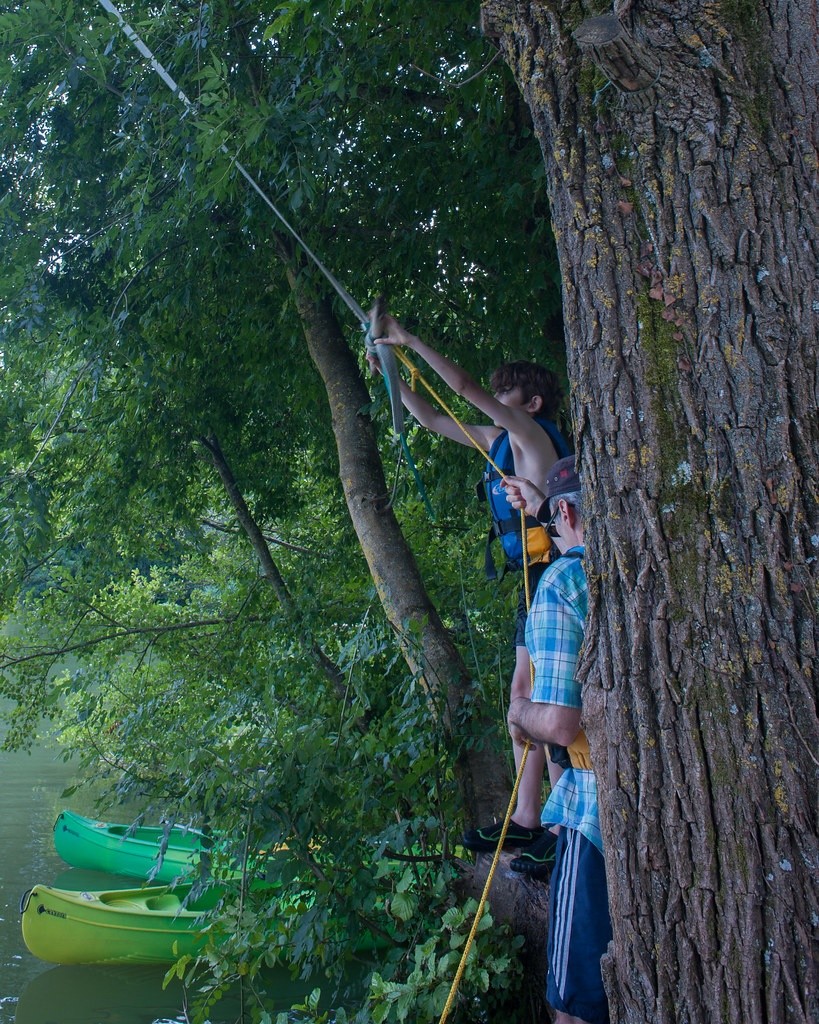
[20, 872, 398, 965]
[52, 808, 472, 886]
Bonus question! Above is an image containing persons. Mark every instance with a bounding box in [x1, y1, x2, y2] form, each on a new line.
[499, 455, 612, 1024]
[364, 308, 574, 872]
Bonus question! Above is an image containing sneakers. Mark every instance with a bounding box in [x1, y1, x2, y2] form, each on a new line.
[461, 818, 544, 851]
[510, 830, 558, 873]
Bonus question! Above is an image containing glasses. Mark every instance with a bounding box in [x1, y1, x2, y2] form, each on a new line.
[495, 382, 514, 392]
[544, 504, 575, 537]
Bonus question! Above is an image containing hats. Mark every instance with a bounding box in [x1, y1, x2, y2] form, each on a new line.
[536, 455, 581, 523]
